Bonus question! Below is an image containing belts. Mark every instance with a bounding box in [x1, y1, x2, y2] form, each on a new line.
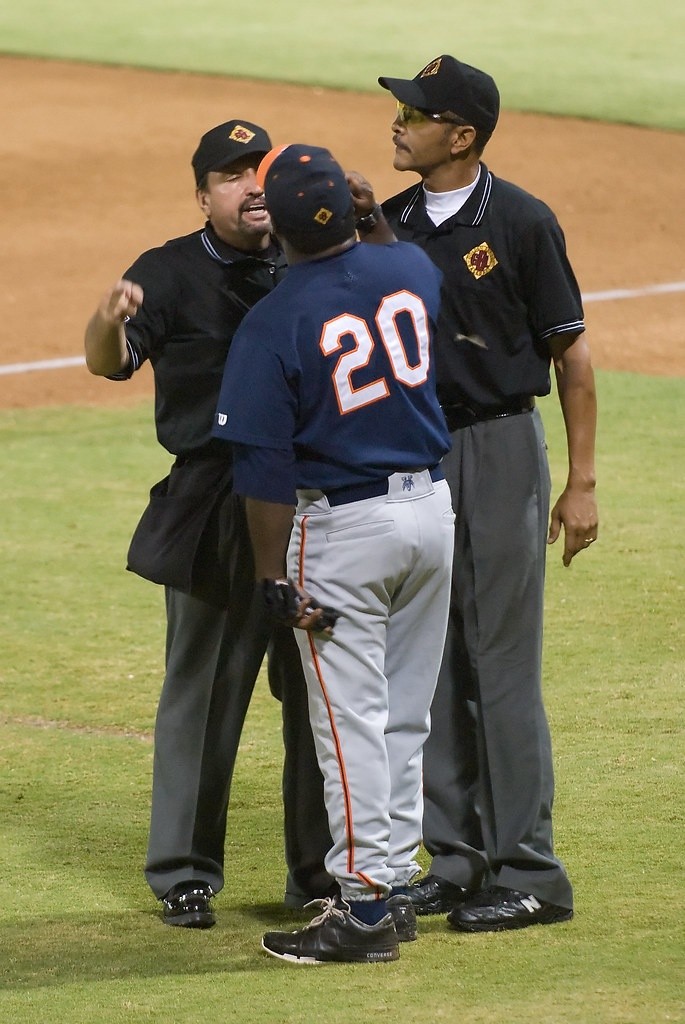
[440, 395, 535, 434]
[322, 463, 445, 507]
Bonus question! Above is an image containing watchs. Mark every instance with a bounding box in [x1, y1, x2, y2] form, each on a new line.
[355, 202, 382, 233]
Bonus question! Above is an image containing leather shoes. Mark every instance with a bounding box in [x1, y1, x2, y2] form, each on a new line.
[162, 880, 216, 926]
[285, 881, 341, 908]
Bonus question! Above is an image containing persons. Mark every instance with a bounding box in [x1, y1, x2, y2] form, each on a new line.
[214, 142, 489, 964]
[88, 121, 345, 928]
[377, 54, 600, 930]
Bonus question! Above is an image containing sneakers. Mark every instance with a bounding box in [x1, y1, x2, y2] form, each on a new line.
[447, 886, 573, 932]
[385, 894, 417, 942]
[261, 894, 400, 962]
[408, 875, 466, 914]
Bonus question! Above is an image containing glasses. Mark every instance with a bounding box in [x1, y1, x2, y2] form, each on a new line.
[397, 100, 477, 128]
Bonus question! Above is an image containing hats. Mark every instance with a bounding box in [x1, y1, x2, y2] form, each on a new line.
[192, 119, 273, 175]
[378, 55, 500, 133]
[256, 143, 350, 230]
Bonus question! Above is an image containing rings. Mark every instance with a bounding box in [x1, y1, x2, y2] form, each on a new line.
[585, 537, 593, 548]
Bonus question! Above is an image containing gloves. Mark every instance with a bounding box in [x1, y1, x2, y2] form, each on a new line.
[262, 574, 339, 630]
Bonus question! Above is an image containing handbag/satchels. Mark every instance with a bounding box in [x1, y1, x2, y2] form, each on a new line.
[126, 484, 221, 598]
